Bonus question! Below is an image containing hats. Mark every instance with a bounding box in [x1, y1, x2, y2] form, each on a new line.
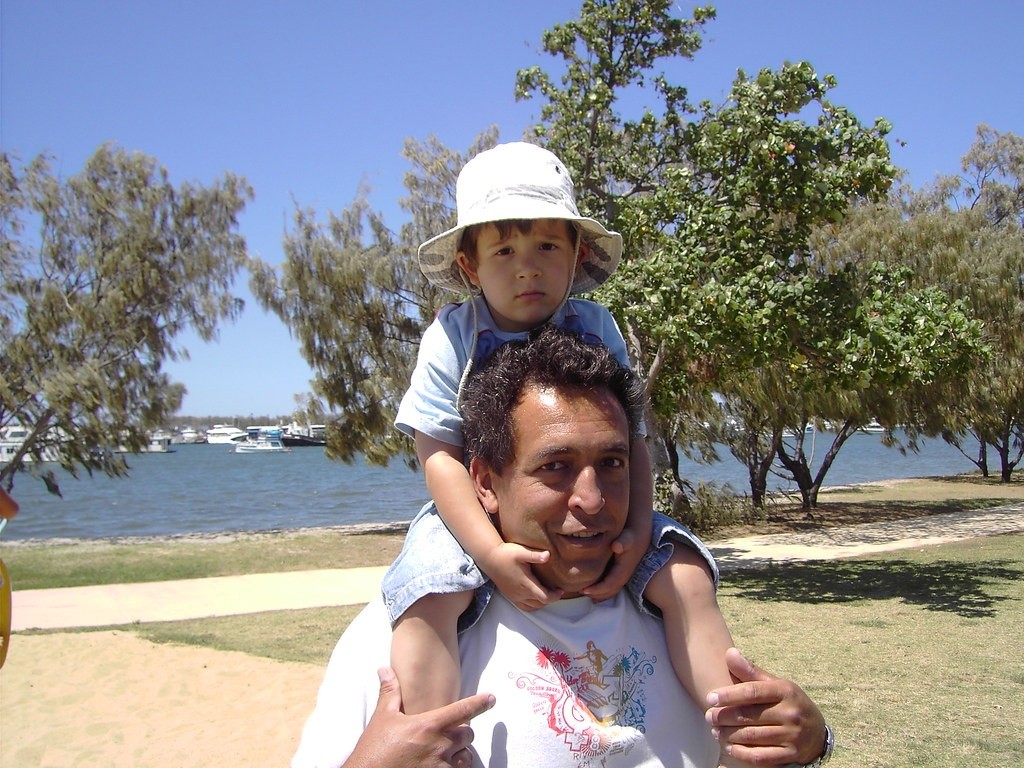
[417, 142, 623, 295]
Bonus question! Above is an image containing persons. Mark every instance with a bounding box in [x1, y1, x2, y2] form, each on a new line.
[379, 142, 745, 768]
[0, 484, 20, 520]
[291, 319, 835, 768]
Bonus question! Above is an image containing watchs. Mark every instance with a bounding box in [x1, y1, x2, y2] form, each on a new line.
[802, 724, 834, 768]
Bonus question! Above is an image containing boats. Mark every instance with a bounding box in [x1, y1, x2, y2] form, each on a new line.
[0, 414, 342, 464]
